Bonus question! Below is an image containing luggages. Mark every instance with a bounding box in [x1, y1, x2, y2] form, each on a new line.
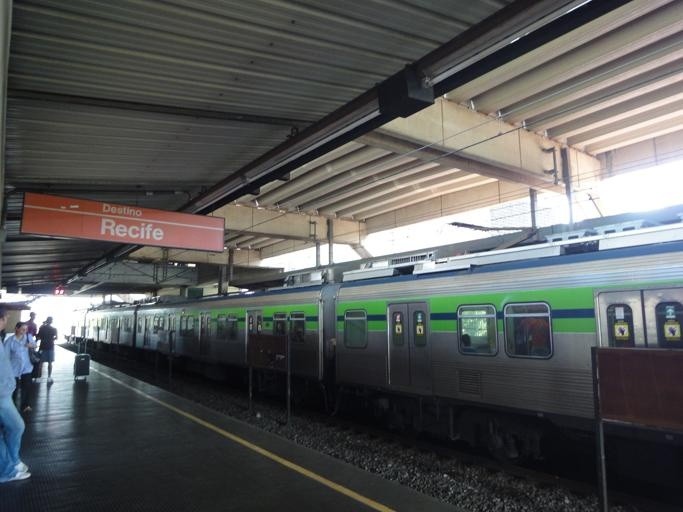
[74, 341, 90, 380]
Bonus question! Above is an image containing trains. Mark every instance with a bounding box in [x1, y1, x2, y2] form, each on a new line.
[63, 204, 682, 476]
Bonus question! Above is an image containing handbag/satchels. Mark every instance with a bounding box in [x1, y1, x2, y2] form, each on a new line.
[26, 332, 40, 363]
[31, 361, 43, 378]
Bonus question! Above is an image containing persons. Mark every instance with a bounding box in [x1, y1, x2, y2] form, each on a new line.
[2, 320, 37, 414]
[23, 312, 37, 361]
[0, 305, 32, 484]
[526, 316, 553, 358]
[460, 333, 480, 353]
[514, 316, 532, 355]
[34, 315, 58, 383]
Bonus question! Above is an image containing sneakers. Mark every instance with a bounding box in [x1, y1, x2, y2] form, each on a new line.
[33, 379, 41, 384]
[47, 377, 54, 383]
[0, 461, 31, 483]
[24, 406, 33, 413]
